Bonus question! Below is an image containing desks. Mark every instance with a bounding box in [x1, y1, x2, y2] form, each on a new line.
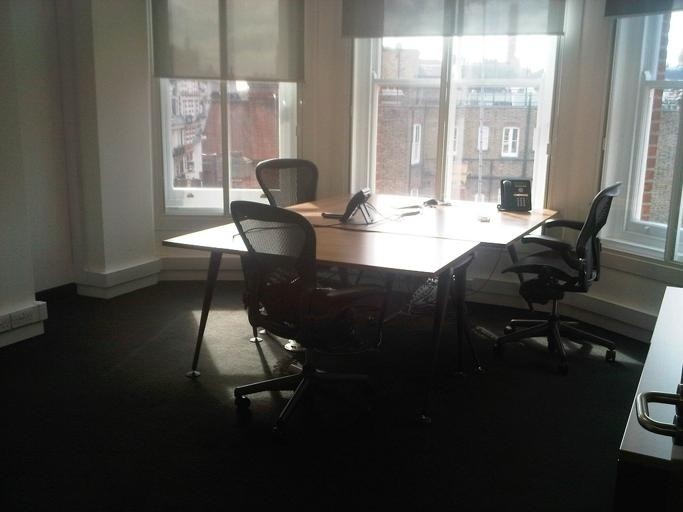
[281, 192, 559, 347]
[613, 284, 682, 510]
[162, 219, 483, 422]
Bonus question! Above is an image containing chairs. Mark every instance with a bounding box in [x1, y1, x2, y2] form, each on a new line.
[257, 160, 354, 352]
[493, 181, 621, 388]
[231, 200, 386, 446]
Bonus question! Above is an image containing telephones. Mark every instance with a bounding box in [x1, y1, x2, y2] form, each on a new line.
[500, 179, 531, 211]
[339, 187, 371, 224]
